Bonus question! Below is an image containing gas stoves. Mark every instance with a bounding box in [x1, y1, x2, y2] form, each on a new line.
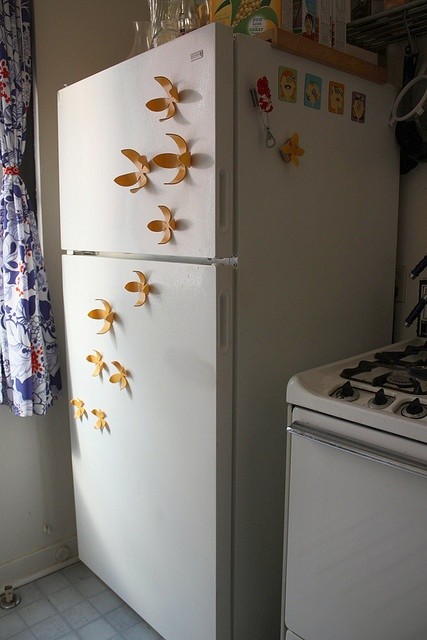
[325, 292, 425, 415]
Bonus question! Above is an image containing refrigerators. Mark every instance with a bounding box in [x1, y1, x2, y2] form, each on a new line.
[30, 24, 391, 635]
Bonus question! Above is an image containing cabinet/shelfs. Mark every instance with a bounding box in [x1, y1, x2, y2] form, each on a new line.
[279, 424, 427, 640]
